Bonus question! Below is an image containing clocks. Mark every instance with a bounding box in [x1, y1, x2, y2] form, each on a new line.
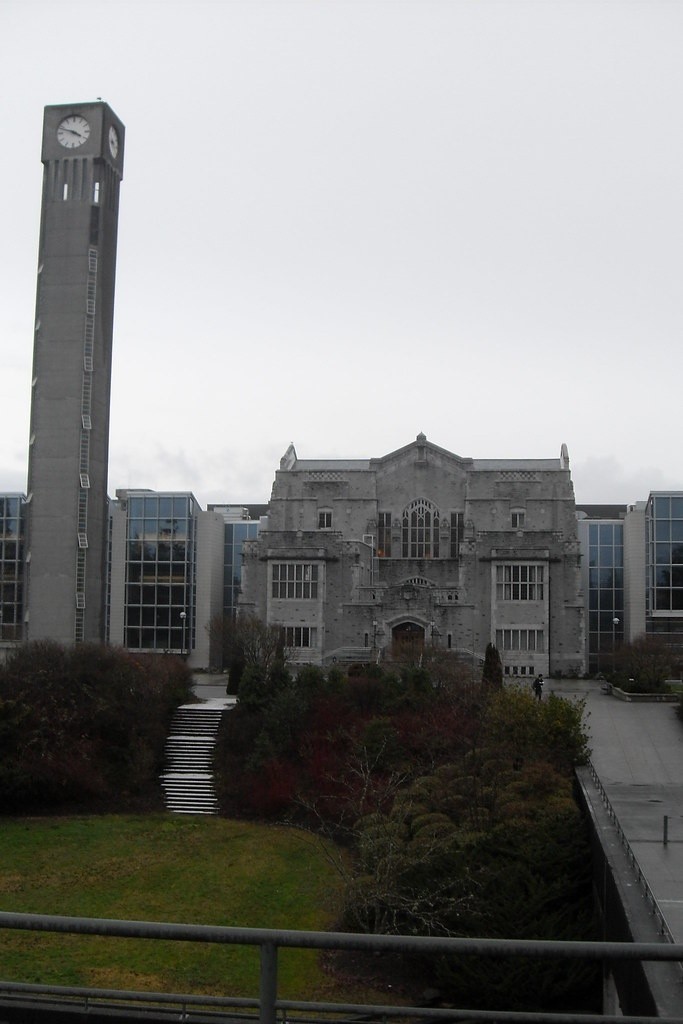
[109, 125, 118, 159]
[57, 114, 90, 149]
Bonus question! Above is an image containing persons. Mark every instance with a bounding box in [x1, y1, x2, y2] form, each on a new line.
[532, 674, 544, 700]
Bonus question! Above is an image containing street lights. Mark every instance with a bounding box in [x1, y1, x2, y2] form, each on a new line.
[179, 610, 186, 659]
[430, 617, 435, 650]
[613, 616, 619, 673]
[372, 617, 377, 662]
